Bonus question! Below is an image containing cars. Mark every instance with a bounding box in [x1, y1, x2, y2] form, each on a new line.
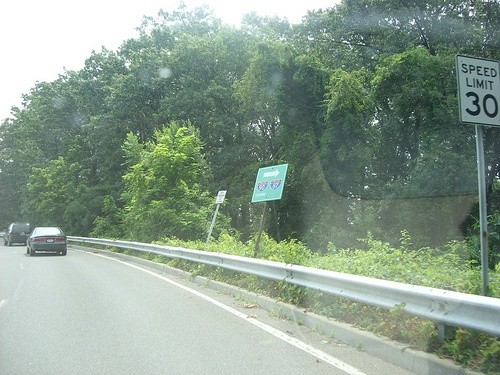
[26, 225, 67, 256]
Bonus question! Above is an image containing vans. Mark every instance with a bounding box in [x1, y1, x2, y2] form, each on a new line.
[3, 222, 30, 245]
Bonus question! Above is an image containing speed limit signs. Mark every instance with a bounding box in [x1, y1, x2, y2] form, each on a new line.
[456, 56, 500, 127]
